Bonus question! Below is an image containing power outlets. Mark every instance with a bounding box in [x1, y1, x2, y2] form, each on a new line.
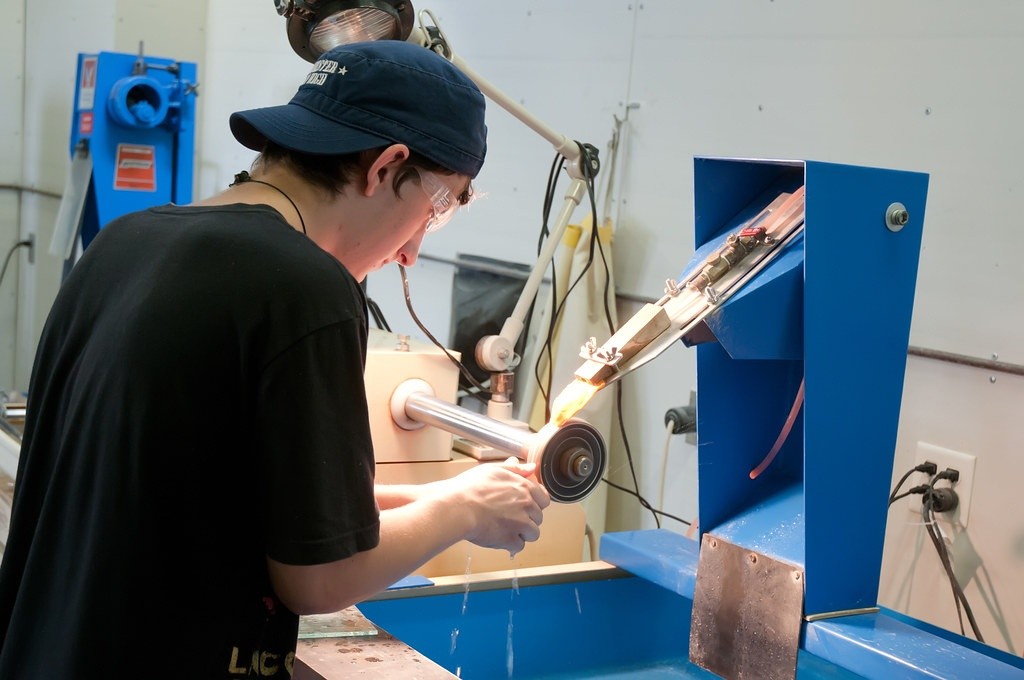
[908, 440, 976, 529]
[28, 232, 36, 264]
[685, 389, 697, 448]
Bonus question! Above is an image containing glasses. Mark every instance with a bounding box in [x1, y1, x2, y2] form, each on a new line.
[411, 163, 461, 232]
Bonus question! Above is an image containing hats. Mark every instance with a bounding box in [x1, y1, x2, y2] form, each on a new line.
[227, 41, 488, 179]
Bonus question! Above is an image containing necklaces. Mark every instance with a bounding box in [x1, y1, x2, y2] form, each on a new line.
[227, 167, 309, 240]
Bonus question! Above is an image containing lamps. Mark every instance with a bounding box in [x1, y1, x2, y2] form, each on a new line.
[272, 0, 603, 463]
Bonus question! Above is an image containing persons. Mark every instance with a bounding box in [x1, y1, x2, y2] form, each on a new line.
[0, 37, 552, 680]
